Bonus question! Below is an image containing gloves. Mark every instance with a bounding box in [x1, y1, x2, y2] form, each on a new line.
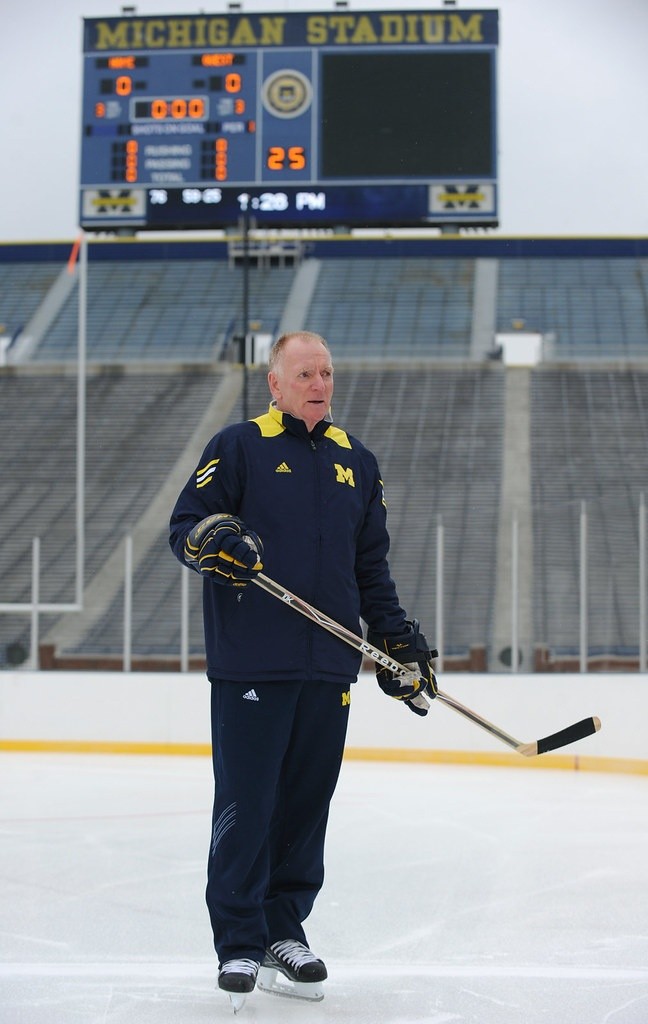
[184, 514, 264, 588]
[367, 618, 438, 716]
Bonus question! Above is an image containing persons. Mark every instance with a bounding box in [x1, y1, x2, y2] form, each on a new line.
[170, 332, 436, 1012]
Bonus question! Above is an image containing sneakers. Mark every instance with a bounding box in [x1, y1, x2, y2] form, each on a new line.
[218, 957, 260, 1015]
[257, 939, 327, 1002]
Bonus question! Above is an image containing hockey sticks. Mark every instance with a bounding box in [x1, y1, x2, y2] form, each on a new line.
[248, 572, 603, 759]
